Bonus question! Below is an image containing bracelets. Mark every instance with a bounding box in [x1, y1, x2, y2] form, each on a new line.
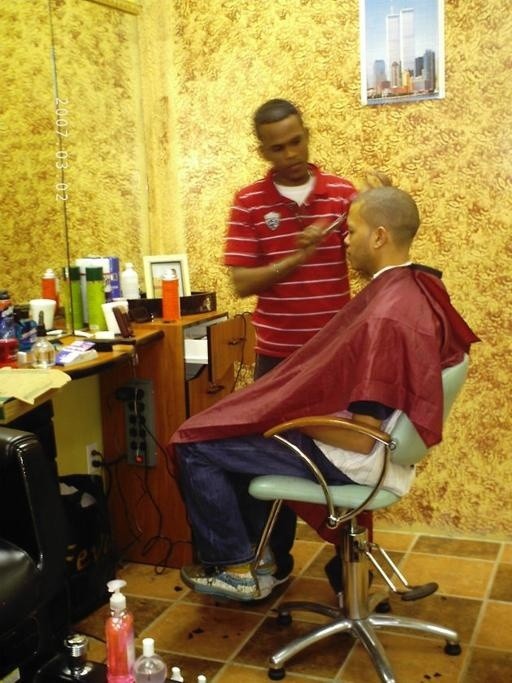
[271, 263, 283, 277]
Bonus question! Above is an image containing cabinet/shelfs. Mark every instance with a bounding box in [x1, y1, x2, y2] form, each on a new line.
[101, 310, 255, 570]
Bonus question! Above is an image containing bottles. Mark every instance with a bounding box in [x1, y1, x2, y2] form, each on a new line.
[41, 266, 61, 316]
[57, 633, 95, 683]
[61, 263, 83, 332]
[160, 266, 182, 323]
[81, 264, 107, 332]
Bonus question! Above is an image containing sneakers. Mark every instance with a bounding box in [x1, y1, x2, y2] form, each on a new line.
[182, 565, 263, 602]
[248, 545, 276, 574]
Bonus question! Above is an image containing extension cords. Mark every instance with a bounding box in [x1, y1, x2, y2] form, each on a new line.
[124, 378, 157, 468]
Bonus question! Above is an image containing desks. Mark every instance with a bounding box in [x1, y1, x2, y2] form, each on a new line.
[0, 322, 163, 424]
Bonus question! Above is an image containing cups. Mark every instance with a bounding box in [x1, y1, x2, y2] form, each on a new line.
[28, 297, 57, 331]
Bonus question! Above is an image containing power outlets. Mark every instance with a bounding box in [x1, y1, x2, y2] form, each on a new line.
[87, 446, 105, 476]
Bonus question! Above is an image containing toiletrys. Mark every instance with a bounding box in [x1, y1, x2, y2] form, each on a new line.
[106, 580, 137, 683]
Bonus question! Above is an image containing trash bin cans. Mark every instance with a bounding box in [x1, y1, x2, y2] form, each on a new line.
[59, 474, 116, 620]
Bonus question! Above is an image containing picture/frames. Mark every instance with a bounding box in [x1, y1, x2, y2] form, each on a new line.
[144, 254, 192, 299]
[358, 0, 444, 106]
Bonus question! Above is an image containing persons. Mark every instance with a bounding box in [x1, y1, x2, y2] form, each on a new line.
[224, 99, 389, 599]
[177, 185, 480, 602]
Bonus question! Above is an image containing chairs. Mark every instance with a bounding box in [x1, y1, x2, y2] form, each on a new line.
[0, 424, 69, 683]
[248, 350, 469, 683]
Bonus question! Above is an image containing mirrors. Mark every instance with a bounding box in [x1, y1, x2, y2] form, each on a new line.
[0, 0, 150, 341]
